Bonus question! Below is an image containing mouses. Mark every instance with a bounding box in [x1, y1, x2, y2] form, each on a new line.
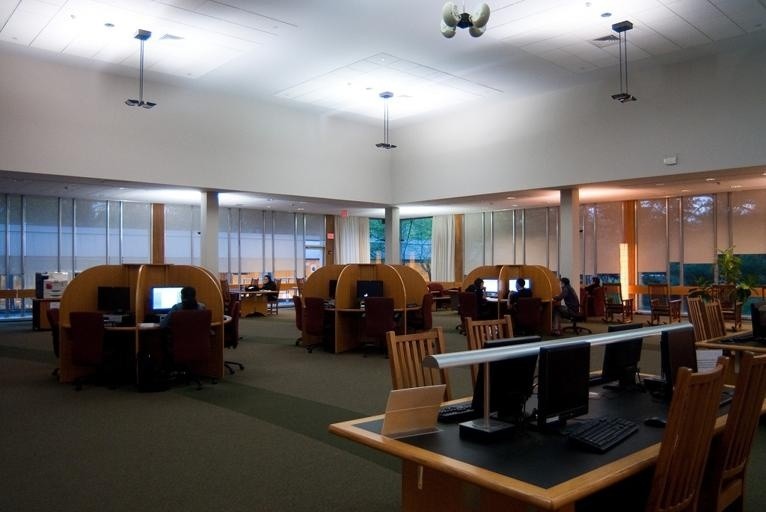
[643, 415, 669, 428]
[721, 337, 734, 343]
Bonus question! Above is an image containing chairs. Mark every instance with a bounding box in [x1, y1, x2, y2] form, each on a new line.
[34, 278, 284, 392]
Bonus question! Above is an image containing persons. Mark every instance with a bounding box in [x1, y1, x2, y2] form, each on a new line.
[474, 278, 490, 302]
[260, 275, 277, 301]
[553, 278, 579, 329]
[584, 277, 600, 294]
[506, 278, 533, 310]
[160, 287, 206, 323]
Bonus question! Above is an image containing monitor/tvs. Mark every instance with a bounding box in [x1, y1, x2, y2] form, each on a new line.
[330, 279, 335, 298]
[601, 323, 648, 395]
[481, 277, 497, 296]
[148, 284, 183, 313]
[96, 286, 130, 310]
[473, 334, 537, 406]
[507, 278, 531, 293]
[356, 280, 385, 298]
[750, 302, 766, 339]
[538, 343, 590, 429]
[659, 328, 698, 388]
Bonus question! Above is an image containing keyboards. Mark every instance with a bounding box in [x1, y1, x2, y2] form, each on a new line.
[438, 400, 477, 422]
[716, 387, 738, 407]
[565, 413, 640, 451]
[590, 371, 602, 387]
[730, 329, 753, 343]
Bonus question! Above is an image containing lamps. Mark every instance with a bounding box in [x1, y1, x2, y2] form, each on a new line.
[440, 0, 490, 38]
[375, 92, 397, 150]
[124, 29, 157, 110]
[610, 21, 637, 103]
